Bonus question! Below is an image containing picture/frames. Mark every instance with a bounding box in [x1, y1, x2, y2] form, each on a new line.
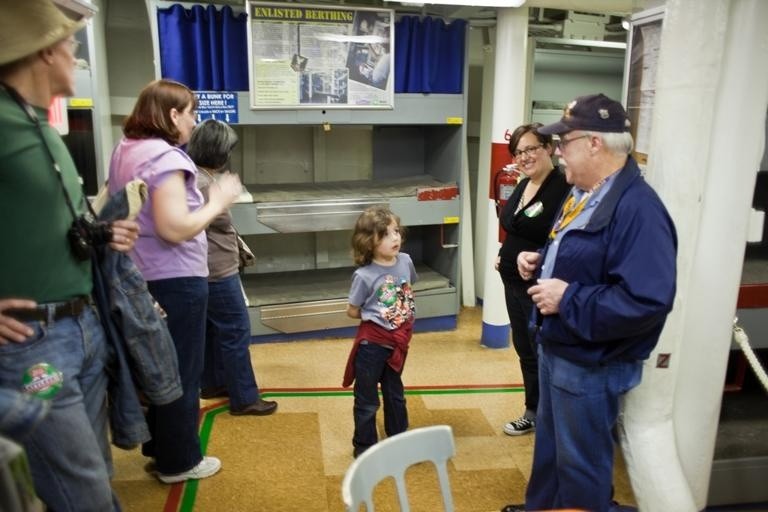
[241, 0, 397, 114]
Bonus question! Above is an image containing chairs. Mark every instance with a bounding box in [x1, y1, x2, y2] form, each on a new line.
[336, 422, 464, 512]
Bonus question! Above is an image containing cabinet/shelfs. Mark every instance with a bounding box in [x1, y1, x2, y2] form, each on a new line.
[98, 1, 472, 338]
[522, 26, 629, 117]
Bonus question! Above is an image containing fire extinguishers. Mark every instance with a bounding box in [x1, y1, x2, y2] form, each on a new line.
[494, 163, 521, 243]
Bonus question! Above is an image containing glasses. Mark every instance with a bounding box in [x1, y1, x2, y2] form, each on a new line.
[514, 144, 547, 157]
[554, 134, 588, 151]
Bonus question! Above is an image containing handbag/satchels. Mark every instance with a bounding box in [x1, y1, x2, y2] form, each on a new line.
[237, 234, 256, 267]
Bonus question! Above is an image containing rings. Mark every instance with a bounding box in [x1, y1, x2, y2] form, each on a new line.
[124, 239, 131, 245]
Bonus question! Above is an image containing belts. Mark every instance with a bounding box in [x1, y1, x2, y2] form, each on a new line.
[0, 293, 93, 322]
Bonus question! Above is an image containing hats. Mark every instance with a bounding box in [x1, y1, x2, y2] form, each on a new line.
[535, 91, 634, 136]
[0, 0, 90, 71]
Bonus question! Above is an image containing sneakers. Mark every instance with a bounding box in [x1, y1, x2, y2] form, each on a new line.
[152, 453, 222, 485]
[500, 415, 538, 436]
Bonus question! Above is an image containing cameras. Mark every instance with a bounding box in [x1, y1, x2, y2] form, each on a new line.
[66, 214, 114, 262]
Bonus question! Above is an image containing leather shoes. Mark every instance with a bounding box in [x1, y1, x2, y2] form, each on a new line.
[200, 383, 232, 399]
[230, 395, 280, 417]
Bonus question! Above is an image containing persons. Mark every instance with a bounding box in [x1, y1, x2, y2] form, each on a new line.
[342, 205, 419, 457]
[499, 89, 676, 511]
[1, 0, 141, 510]
[500, 121, 574, 436]
[183, 117, 278, 416]
[106, 77, 244, 485]
